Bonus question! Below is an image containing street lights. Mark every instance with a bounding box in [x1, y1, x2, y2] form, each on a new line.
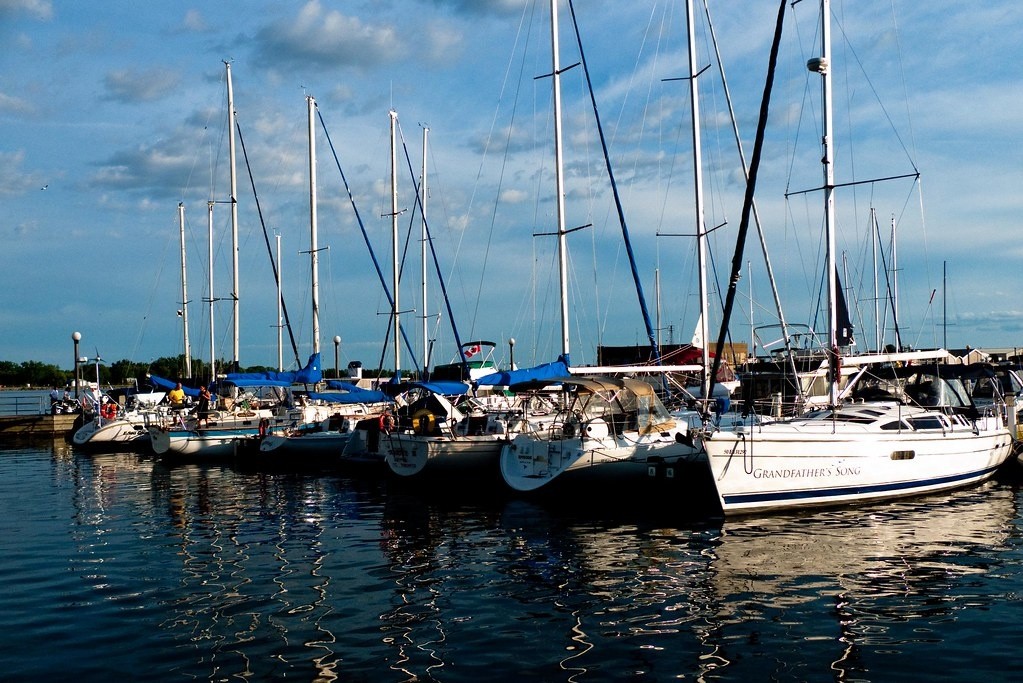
[509, 337, 515, 371]
[71, 331, 87, 398]
[333, 335, 342, 378]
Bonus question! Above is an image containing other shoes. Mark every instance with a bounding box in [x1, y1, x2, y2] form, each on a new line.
[197, 425, 201, 429]
[206, 424, 209, 428]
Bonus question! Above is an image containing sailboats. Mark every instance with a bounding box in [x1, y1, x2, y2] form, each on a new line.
[72, 1, 1023, 519]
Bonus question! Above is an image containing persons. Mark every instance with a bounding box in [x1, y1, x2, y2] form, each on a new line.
[49, 386, 58, 413]
[62, 387, 71, 400]
[196, 384, 211, 429]
[168, 383, 187, 427]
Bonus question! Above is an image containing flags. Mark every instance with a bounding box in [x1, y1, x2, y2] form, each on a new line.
[464, 345, 481, 358]
